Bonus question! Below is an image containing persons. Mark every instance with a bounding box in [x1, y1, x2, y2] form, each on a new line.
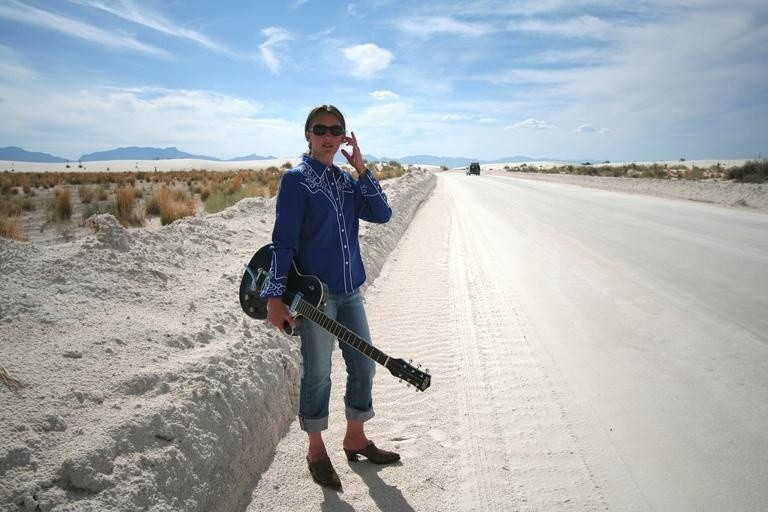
[260, 105, 400, 488]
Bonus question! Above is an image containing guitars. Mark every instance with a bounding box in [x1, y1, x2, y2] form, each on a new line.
[240, 243, 431, 392]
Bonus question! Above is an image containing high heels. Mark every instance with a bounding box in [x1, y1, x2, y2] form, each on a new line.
[343, 440, 401, 464]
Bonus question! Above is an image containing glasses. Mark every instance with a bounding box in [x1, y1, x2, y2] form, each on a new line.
[308, 124, 343, 137]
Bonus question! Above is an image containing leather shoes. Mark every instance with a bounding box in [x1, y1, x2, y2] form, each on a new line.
[306, 453, 342, 489]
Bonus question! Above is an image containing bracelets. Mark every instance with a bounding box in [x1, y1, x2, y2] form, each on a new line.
[359, 167, 367, 177]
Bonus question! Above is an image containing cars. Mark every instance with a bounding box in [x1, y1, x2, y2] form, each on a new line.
[466, 162, 480, 175]
